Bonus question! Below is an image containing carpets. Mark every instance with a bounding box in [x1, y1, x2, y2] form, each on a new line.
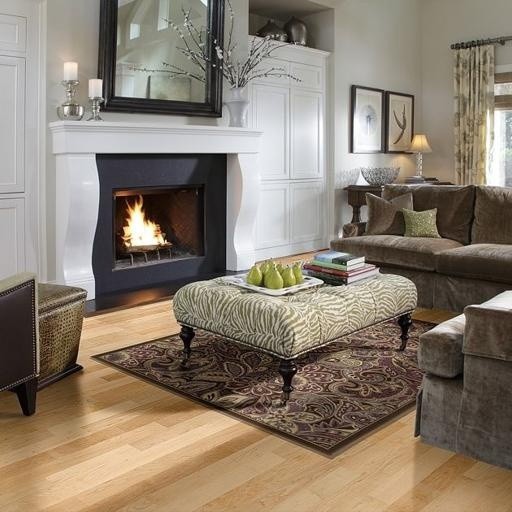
[90, 318, 441, 454]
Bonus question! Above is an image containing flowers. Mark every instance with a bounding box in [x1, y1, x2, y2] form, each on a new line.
[128, 5, 307, 87]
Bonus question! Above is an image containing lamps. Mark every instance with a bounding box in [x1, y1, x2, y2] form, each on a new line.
[404, 134, 439, 184]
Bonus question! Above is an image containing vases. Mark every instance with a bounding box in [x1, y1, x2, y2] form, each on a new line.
[223, 83, 254, 126]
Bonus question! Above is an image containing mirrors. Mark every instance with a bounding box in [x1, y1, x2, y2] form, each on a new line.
[97, 1, 225, 118]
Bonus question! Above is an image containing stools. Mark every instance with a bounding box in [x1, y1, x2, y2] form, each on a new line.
[37, 282, 88, 393]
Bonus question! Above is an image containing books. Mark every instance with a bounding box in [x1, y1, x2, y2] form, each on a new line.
[300, 249, 381, 287]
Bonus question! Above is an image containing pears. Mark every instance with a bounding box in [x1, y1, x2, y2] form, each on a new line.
[248, 259, 303, 290]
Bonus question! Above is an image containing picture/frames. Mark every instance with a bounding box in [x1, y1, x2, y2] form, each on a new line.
[349, 85, 416, 155]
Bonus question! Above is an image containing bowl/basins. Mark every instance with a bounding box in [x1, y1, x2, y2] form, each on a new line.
[360, 167, 401, 187]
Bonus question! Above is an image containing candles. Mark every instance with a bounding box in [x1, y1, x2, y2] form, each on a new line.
[61, 60, 80, 83]
[87, 76, 104, 99]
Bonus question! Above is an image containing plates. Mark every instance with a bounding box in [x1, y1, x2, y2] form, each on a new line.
[222, 275, 324, 296]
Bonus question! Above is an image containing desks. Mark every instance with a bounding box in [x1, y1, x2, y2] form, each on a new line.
[168, 254, 419, 400]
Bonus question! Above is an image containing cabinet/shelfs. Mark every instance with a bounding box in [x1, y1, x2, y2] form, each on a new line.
[250, 39, 330, 267]
